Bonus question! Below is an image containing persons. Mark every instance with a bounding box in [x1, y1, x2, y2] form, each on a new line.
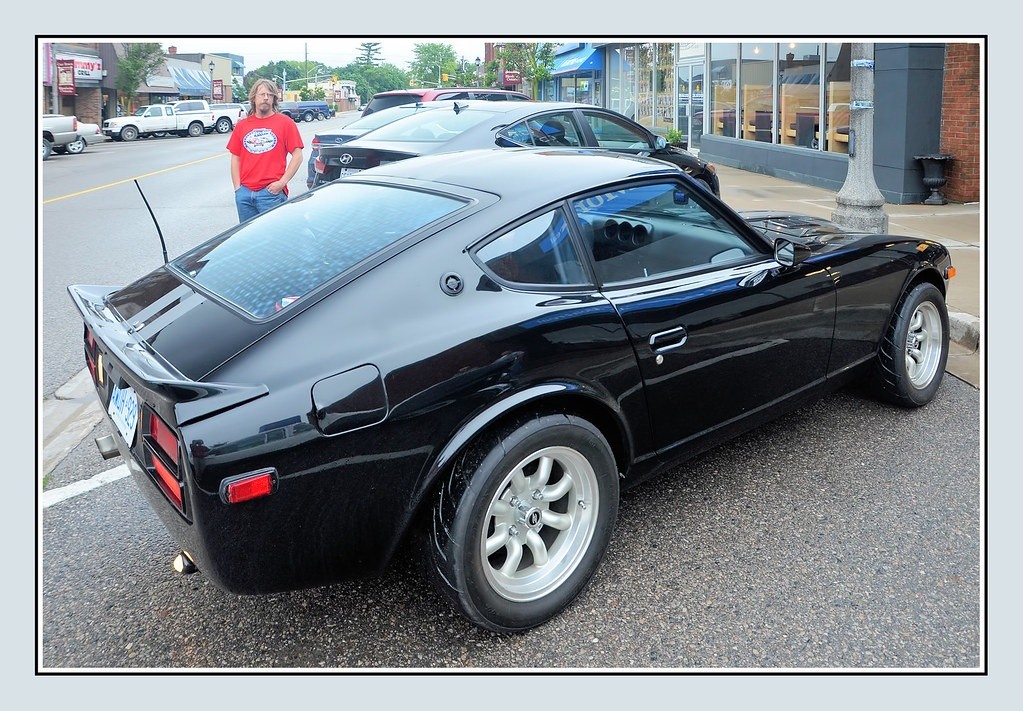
[225, 79, 305, 224]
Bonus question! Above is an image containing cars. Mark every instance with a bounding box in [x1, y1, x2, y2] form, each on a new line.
[309, 99, 723, 215]
[209, 100, 336, 125]
[303, 99, 544, 190]
[50, 120, 105, 156]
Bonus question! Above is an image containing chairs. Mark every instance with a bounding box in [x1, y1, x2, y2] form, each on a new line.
[535, 120, 571, 146]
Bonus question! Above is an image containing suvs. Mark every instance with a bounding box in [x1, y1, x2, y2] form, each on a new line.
[694, 93, 831, 134]
[359, 87, 538, 119]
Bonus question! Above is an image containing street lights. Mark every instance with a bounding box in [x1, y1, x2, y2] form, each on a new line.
[208, 60, 216, 104]
[475, 56, 481, 88]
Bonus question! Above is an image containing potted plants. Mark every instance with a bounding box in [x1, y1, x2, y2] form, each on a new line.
[169, 45, 177, 54]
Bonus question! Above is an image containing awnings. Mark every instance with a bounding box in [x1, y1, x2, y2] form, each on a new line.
[523, 48, 604, 80]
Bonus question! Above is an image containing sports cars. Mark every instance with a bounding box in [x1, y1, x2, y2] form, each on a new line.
[66, 141, 955, 637]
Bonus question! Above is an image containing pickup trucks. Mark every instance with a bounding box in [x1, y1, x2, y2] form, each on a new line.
[101, 104, 215, 142]
[150, 98, 244, 138]
[43, 114, 80, 161]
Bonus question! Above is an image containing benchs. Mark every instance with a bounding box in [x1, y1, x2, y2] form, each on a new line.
[715, 81, 850, 154]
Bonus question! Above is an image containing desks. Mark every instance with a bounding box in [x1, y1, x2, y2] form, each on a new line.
[755, 110, 781, 142]
[795, 111, 828, 149]
[722, 109, 744, 138]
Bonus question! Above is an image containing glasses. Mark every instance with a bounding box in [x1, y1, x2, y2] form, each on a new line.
[256, 93, 274, 99]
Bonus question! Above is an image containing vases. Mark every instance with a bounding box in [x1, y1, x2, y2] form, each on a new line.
[913, 153, 954, 206]
